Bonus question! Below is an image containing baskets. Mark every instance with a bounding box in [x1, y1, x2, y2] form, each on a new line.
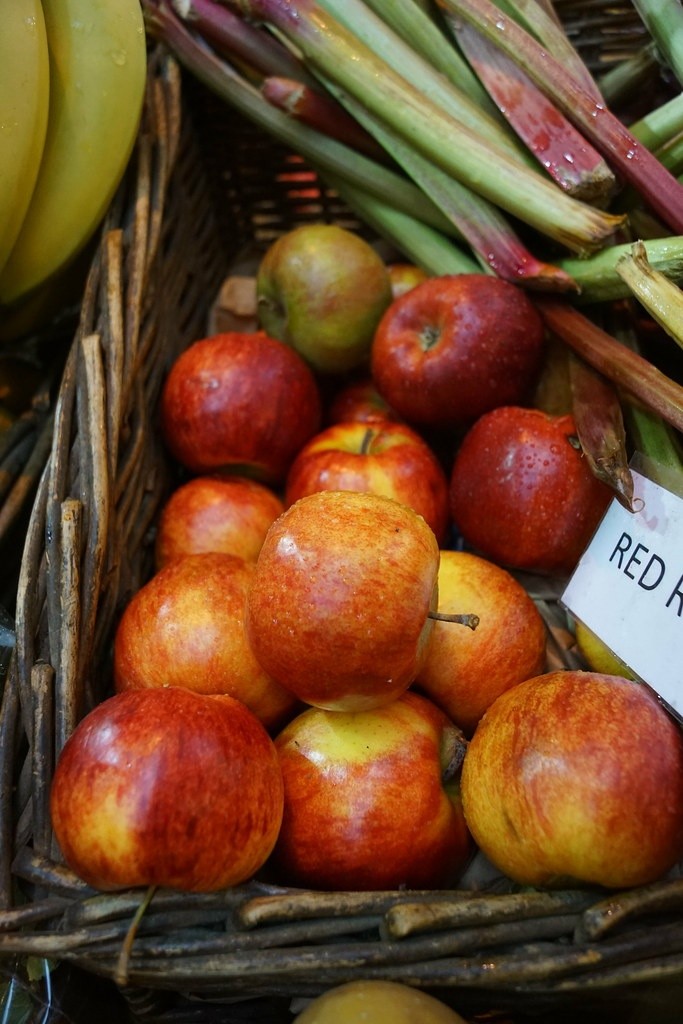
[0, 0, 683, 1024]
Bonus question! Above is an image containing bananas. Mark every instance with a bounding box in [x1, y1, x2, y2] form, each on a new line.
[0, 0, 146, 306]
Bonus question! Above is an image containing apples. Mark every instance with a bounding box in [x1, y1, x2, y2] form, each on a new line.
[52, 223, 683, 1024]
